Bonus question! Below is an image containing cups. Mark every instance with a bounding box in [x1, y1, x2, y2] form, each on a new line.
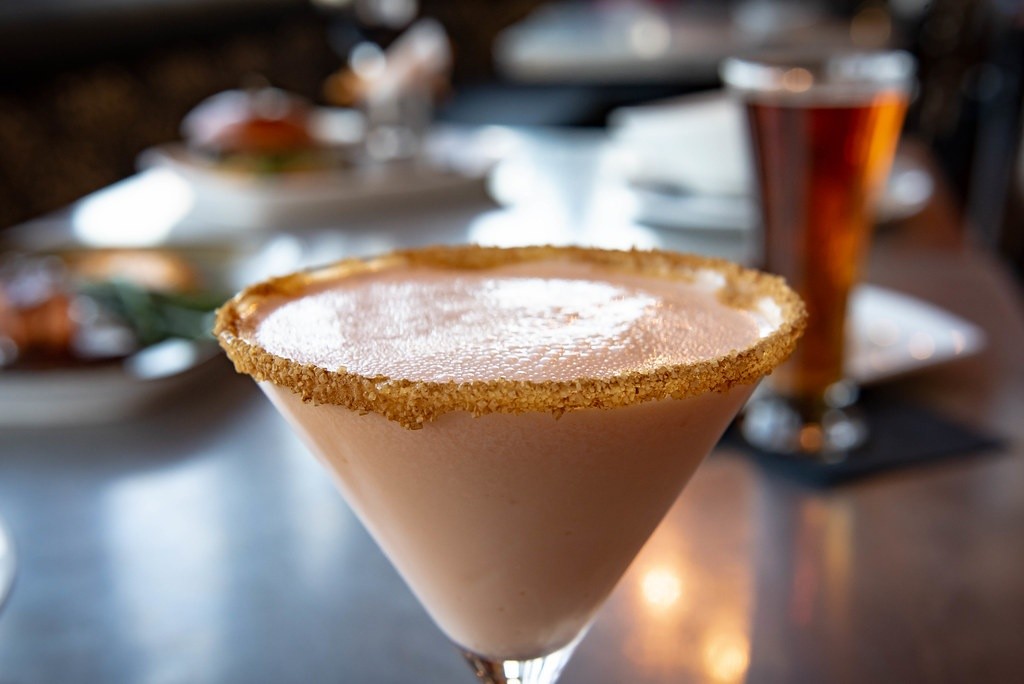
[721, 41, 913, 457]
[213, 246, 809, 684]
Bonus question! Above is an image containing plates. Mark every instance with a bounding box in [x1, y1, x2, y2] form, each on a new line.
[0, 339, 220, 425]
[852, 286, 988, 386]
[607, 158, 930, 225]
[153, 141, 494, 200]
